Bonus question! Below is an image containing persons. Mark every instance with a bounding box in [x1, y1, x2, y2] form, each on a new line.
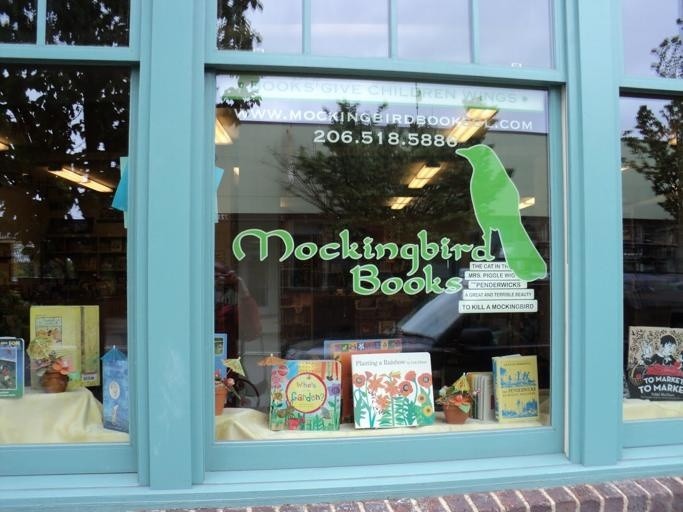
[650, 335, 678, 367]
[213, 259, 263, 363]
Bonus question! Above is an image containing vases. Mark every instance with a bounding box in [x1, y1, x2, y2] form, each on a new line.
[38, 371, 69, 394]
[443, 404, 470, 424]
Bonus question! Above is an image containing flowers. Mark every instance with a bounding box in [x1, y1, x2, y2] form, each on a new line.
[26, 335, 78, 379]
[435, 386, 481, 412]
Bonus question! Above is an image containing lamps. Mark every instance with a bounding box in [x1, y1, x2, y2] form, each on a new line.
[48, 166, 112, 197]
[407, 161, 441, 189]
[214, 116, 236, 147]
[446, 106, 500, 145]
[1, 139, 16, 152]
[390, 198, 413, 210]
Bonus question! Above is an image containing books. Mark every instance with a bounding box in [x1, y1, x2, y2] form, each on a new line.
[0, 336, 25, 399]
[103, 359, 129, 434]
[215, 333, 228, 380]
[29, 306, 82, 391]
[269, 359, 342, 432]
[81, 305, 100, 387]
[464, 371, 500, 421]
[490, 354, 540, 422]
[628, 325, 683, 367]
[351, 351, 436, 430]
[324, 339, 402, 424]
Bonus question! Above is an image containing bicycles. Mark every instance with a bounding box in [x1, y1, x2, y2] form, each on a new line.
[227, 352, 260, 411]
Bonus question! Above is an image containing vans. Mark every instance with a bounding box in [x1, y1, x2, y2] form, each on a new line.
[285, 273, 681, 412]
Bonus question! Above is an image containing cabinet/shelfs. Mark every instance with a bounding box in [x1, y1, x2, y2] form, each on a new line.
[35, 218, 127, 302]
[279, 213, 386, 346]
